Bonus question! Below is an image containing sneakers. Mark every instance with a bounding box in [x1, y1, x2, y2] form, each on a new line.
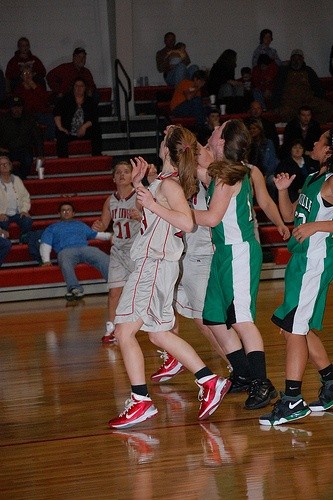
[306, 376, 333, 413]
[65, 288, 86, 301]
[101, 321, 118, 342]
[151, 349, 183, 381]
[258, 391, 312, 427]
[244, 377, 278, 410]
[227, 372, 256, 393]
[108, 392, 158, 429]
[192, 374, 232, 420]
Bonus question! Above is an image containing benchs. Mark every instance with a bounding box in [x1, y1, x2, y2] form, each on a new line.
[0, 77, 333, 302]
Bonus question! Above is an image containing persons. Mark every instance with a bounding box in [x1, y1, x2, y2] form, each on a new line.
[40, 203, 110, 300]
[164, 118, 292, 411]
[156, 28, 333, 202]
[109, 129, 231, 429]
[0, 37, 103, 176]
[259, 134, 333, 425]
[89, 163, 232, 382]
[0, 156, 32, 265]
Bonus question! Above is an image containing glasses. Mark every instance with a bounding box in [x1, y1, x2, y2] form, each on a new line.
[60, 208, 72, 214]
[22, 71, 33, 75]
[0, 162, 11, 167]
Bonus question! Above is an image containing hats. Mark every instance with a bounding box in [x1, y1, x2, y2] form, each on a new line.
[73, 48, 88, 56]
[291, 48, 304, 57]
[8, 96, 25, 107]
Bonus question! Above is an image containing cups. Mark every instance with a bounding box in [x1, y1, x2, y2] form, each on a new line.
[210, 95, 215, 104]
[221, 105, 226, 115]
[36, 158, 43, 171]
[279, 134, 283, 145]
[38, 167, 44, 179]
[140, 76, 148, 86]
[134, 79, 137, 87]
